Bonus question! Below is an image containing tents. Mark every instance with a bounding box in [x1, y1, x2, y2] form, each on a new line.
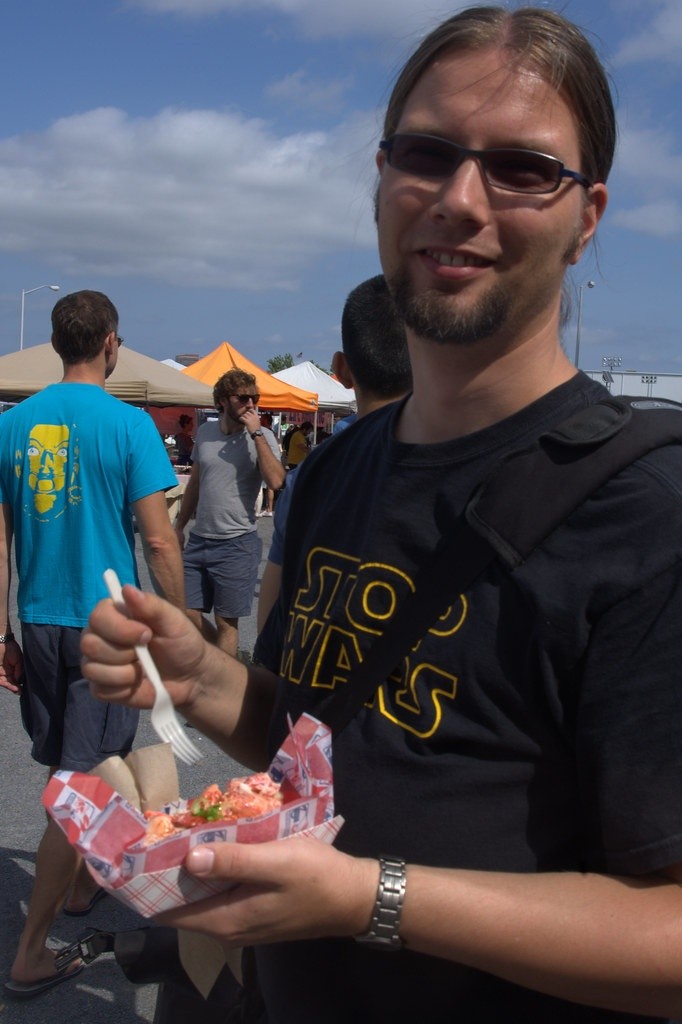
[268, 360, 357, 439]
[145, 341, 319, 444]
[0, 342, 220, 407]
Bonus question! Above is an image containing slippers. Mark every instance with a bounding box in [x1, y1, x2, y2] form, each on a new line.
[60, 886, 107, 917]
[6, 947, 85, 997]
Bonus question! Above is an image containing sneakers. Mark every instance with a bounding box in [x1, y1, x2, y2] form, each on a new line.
[255, 511, 273, 518]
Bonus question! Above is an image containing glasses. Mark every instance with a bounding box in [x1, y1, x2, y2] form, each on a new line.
[229, 393, 260, 405]
[116, 333, 125, 348]
[377, 129, 589, 196]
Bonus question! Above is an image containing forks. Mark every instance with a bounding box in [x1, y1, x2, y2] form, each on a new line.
[105, 569, 204, 766]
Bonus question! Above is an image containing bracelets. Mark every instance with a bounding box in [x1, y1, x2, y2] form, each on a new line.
[0, 633, 15, 643]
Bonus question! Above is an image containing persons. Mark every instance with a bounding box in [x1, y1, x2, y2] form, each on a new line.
[174, 368, 289, 666]
[0, 287, 188, 995]
[176, 412, 345, 519]
[258, 275, 413, 636]
[77, 5, 682, 1024]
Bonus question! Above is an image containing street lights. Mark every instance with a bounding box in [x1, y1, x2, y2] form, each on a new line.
[576, 282, 596, 369]
[602, 356, 622, 371]
[603, 373, 614, 391]
[19, 284, 61, 349]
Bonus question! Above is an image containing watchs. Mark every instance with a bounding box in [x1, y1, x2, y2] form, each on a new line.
[358, 854, 407, 971]
[251, 430, 264, 440]
